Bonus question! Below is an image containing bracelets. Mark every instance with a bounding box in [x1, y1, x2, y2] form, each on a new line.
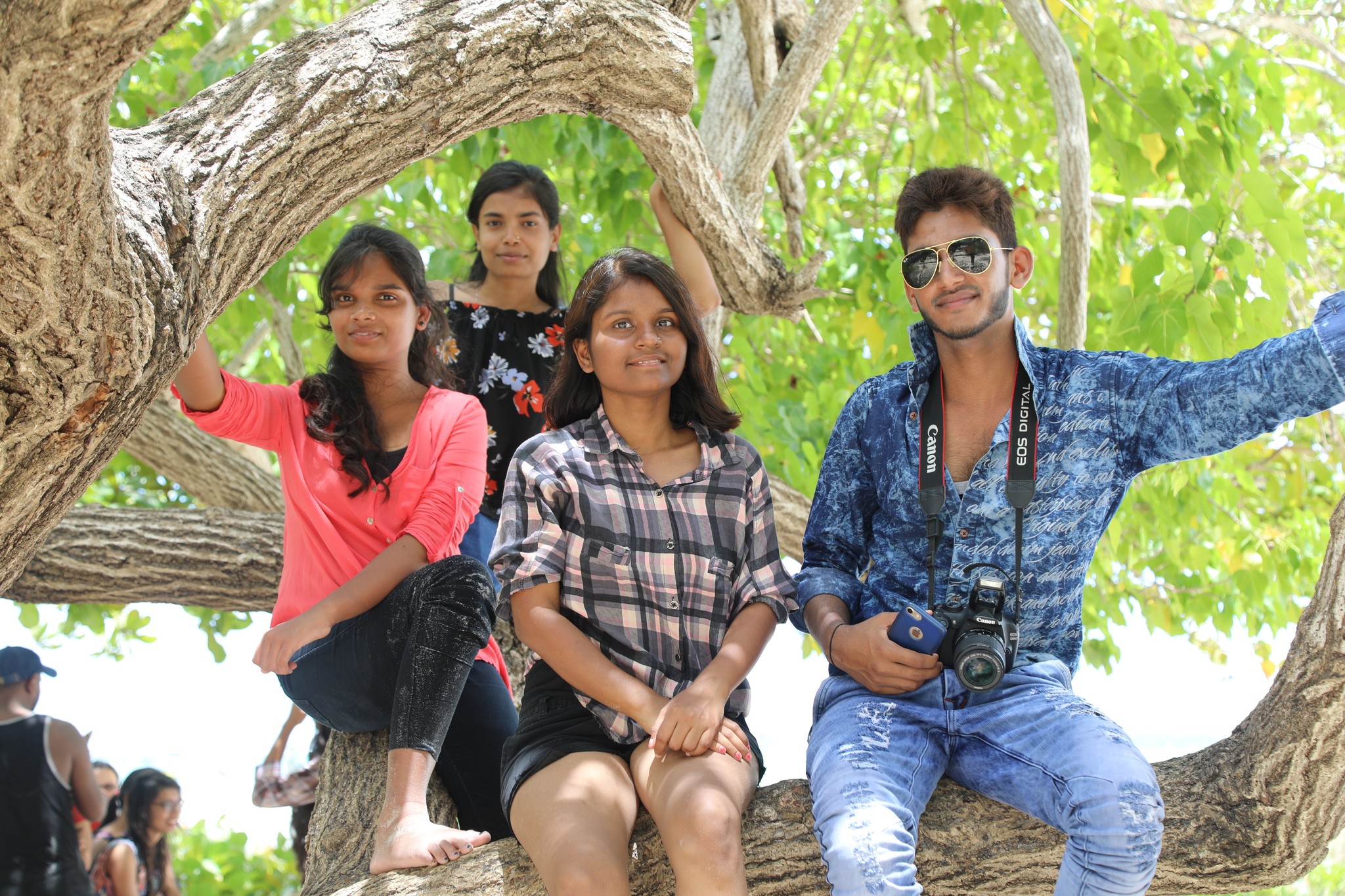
[829, 624, 849, 668]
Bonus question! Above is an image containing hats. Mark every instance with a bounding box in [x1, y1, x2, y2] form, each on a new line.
[0, 646, 57, 686]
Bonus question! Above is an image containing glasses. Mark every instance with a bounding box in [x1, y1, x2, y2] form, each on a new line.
[99, 783, 120, 793]
[899, 235, 1014, 289]
[152, 799, 185, 814]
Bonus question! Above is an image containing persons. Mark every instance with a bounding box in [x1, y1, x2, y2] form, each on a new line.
[421, 160, 724, 592]
[788, 167, 1345, 896]
[252, 703, 332, 888]
[0, 646, 180, 896]
[488, 246, 799, 896]
[178, 224, 520, 872]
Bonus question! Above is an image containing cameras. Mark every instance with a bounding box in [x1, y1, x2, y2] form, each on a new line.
[928, 576, 1020, 695]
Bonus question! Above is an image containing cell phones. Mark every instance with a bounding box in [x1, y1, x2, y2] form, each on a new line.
[889, 602, 949, 654]
